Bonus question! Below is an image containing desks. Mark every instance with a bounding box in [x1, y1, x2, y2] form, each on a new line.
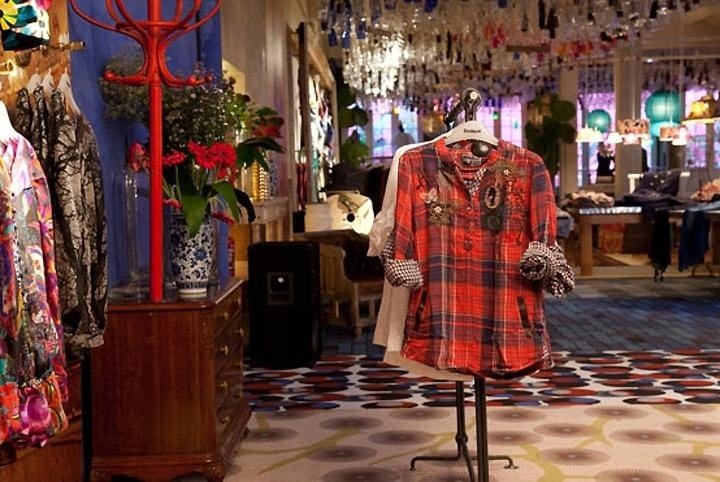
[567, 205, 720, 277]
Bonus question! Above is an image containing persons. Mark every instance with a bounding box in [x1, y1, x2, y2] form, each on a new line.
[596, 143, 615, 176]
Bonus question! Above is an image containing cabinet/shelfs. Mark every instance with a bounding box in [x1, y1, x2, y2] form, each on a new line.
[90, 279, 252, 482]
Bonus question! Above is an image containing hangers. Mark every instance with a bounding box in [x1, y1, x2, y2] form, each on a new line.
[26, 46, 80, 114]
[444, 87, 499, 146]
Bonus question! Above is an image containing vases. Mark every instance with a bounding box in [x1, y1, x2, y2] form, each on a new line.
[168, 216, 217, 299]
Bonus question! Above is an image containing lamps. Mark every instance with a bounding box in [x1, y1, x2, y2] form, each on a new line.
[576, 27, 720, 147]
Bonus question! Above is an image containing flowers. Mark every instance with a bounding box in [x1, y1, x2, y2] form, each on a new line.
[100, 41, 286, 238]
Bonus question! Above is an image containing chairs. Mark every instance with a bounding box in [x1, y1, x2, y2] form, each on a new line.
[320, 244, 383, 339]
